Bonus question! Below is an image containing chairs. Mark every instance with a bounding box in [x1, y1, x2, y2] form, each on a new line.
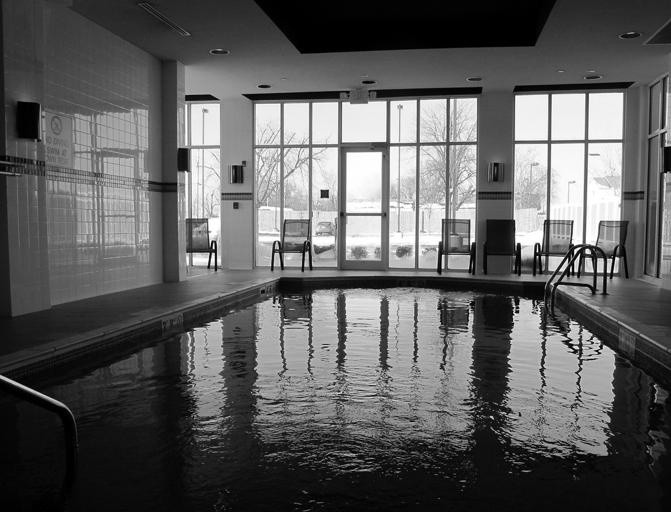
[185, 217, 218, 271]
[270, 218, 313, 273]
[437, 218, 522, 276]
[532, 219, 631, 280]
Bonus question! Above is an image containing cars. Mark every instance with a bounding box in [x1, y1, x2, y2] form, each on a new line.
[315, 222, 335, 235]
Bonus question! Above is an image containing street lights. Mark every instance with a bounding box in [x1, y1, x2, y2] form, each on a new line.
[201, 108, 209, 219]
[530, 163, 539, 184]
[397, 104, 402, 233]
[567, 181, 576, 204]
